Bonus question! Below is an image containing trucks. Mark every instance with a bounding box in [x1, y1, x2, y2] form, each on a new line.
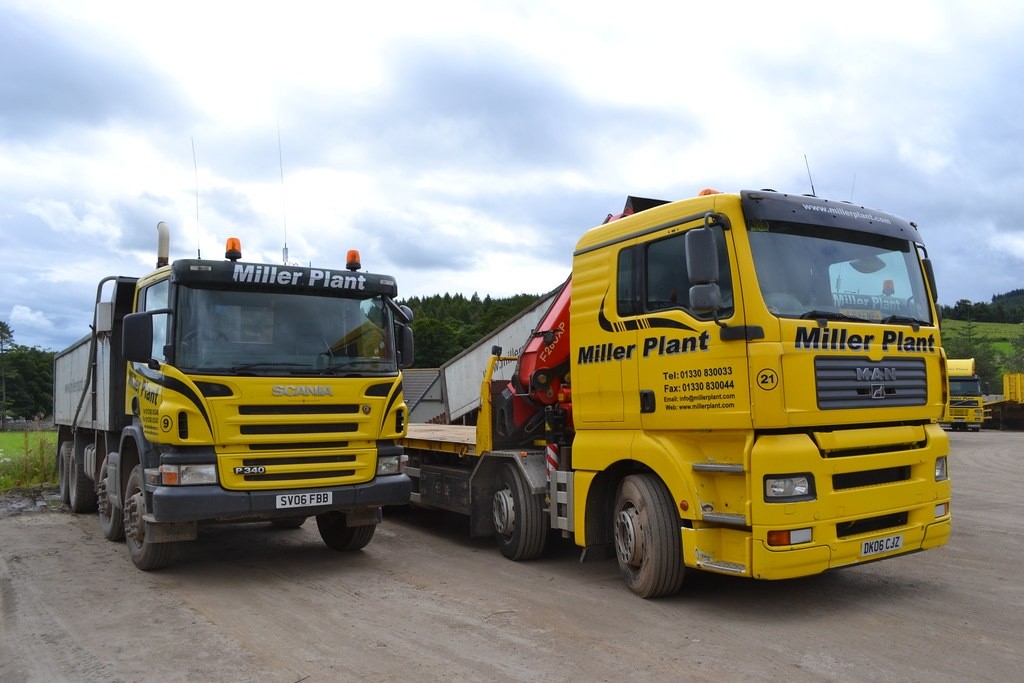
[53, 221, 416, 573]
[946, 357, 985, 431]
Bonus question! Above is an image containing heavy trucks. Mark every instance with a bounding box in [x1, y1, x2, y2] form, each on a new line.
[398, 189, 949, 601]
